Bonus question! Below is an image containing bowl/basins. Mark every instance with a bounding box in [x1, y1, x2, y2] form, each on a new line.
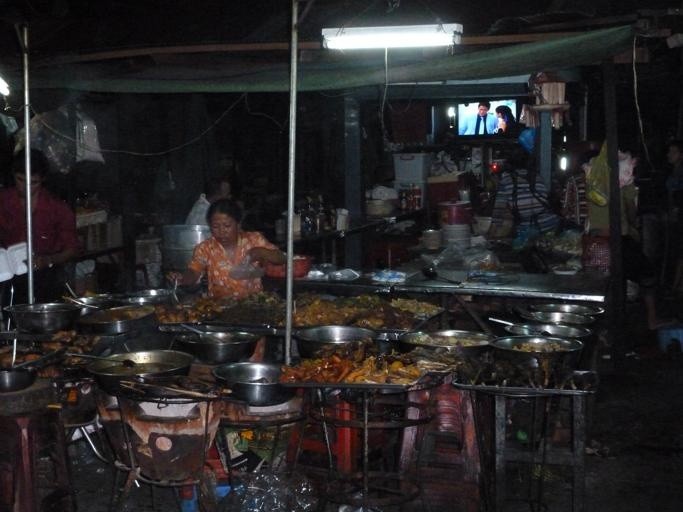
[85, 350, 191, 398]
[179, 331, 261, 366]
[487, 301, 605, 385]
[0, 287, 185, 344]
[291, 326, 375, 363]
[209, 361, 296, 406]
[367, 329, 407, 354]
[1, 369, 33, 391]
[263, 253, 312, 277]
[399, 329, 490, 382]
[469, 215, 514, 239]
[250, 327, 294, 360]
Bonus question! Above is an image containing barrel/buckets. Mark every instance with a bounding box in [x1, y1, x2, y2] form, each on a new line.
[438, 200, 478, 253]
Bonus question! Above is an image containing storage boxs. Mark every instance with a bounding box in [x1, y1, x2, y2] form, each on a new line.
[390, 151, 435, 210]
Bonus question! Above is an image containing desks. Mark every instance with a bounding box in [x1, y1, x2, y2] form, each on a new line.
[267, 214, 618, 336]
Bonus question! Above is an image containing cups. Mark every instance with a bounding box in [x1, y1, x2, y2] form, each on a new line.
[422, 230, 440, 249]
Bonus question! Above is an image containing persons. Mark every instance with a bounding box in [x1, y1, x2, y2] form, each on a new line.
[588, 183, 678, 330]
[164, 197, 287, 300]
[457, 102, 498, 135]
[0, 146, 77, 306]
[490, 148, 551, 225]
[559, 150, 600, 228]
[494, 105, 515, 134]
[663, 142, 683, 291]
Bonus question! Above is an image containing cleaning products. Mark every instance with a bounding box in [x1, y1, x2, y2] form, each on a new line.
[315, 195, 329, 234]
[154, 222, 213, 275]
[306, 196, 315, 213]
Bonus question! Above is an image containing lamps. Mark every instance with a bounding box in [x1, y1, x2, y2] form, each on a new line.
[322, 1, 463, 53]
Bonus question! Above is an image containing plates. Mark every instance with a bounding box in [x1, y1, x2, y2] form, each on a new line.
[443, 223, 472, 252]
[551, 265, 578, 276]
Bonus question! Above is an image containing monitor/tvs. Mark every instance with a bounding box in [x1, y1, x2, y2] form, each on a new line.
[455, 98, 519, 141]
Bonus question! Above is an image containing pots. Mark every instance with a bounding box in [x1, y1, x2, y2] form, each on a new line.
[437, 197, 474, 224]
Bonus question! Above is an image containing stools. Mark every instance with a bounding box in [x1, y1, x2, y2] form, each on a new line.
[397, 380, 478, 485]
[1, 410, 77, 510]
[287, 389, 386, 477]
[186, 441, 243, 502]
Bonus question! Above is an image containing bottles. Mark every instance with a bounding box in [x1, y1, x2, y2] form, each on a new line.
[398, 182, 421, 211]
[297, 194, 326, 234]
[74, 192, 88, 209]
